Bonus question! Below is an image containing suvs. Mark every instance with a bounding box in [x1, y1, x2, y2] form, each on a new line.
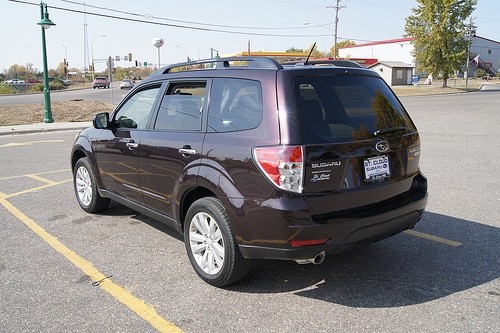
[70, 56, 429, 289]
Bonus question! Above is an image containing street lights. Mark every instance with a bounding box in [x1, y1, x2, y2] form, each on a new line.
[176, 46, 187, 62]
[304, 22, 337, 60]
[91, 35, 105, 73]
[35, 0, 56, 123]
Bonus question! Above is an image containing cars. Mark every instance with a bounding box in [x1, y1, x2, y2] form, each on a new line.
[93, 76, 110, 89]
[4, 74, 75, 86]
[120, 79, 134, 90]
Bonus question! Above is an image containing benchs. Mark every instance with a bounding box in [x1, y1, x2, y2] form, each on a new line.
[413, 79, 431, 88]
